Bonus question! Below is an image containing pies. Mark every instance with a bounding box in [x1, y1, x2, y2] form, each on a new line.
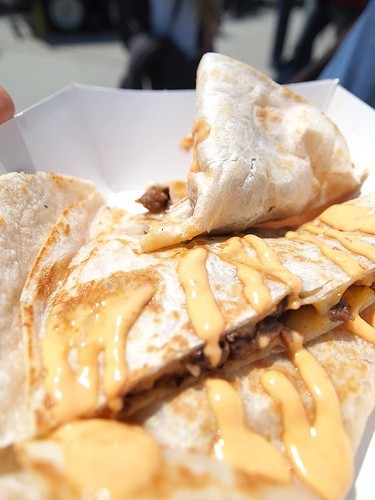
[0, 52, 375, 498]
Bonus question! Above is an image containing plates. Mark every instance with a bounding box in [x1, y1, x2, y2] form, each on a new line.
[0, 76, 375, 497]
